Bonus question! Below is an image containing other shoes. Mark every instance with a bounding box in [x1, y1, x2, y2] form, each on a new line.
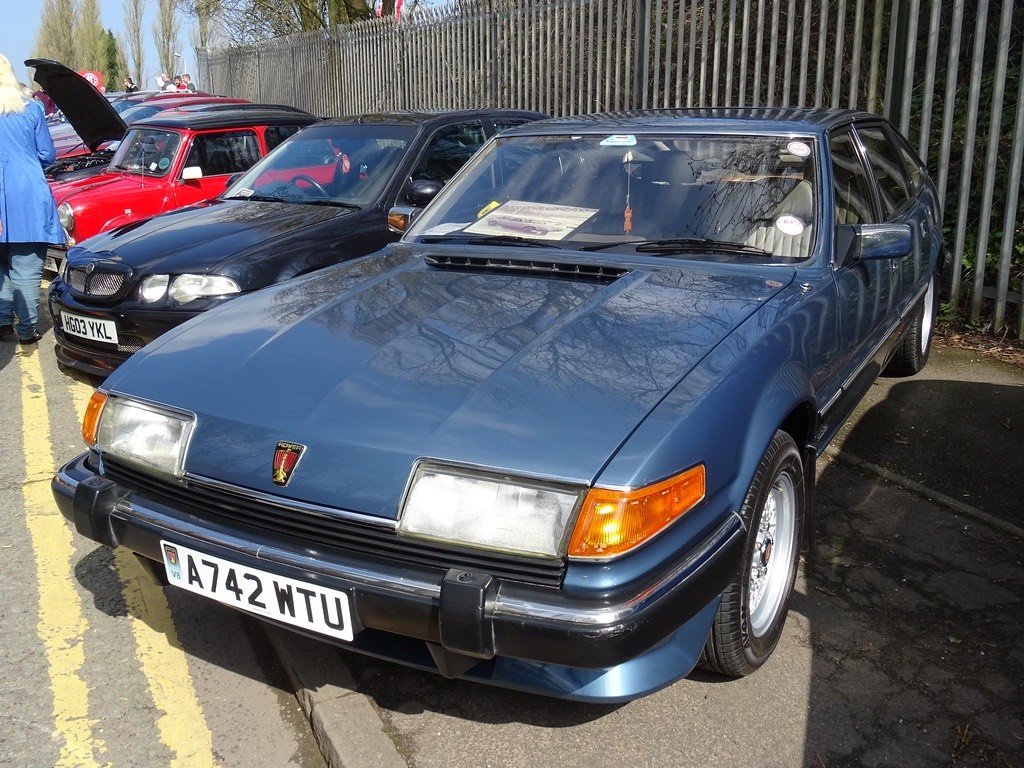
[0, 328, 17, 340]
[20, 331, 42, 344]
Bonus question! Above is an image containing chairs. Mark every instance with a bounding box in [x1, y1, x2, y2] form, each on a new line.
[642, 151, 699, 237]
[578, 176, 662, 237]
[685, 177, 814, 260]
[346, 146, 410, 207]
[207, 149, 232, 174]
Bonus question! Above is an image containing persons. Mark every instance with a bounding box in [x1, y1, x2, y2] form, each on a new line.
[0, 52, 67, 344]
[123, 78, 138, 92]
[161, 73, 195, 93]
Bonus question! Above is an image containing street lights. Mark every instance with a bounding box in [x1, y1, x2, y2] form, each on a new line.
[174, 53, 187, 75]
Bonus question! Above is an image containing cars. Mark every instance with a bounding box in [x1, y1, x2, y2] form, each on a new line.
[50, 103, 943, 706]
[50, 106, 675, 391]
[23, 57, 256, 191]
[45, 111, 356, 275]
